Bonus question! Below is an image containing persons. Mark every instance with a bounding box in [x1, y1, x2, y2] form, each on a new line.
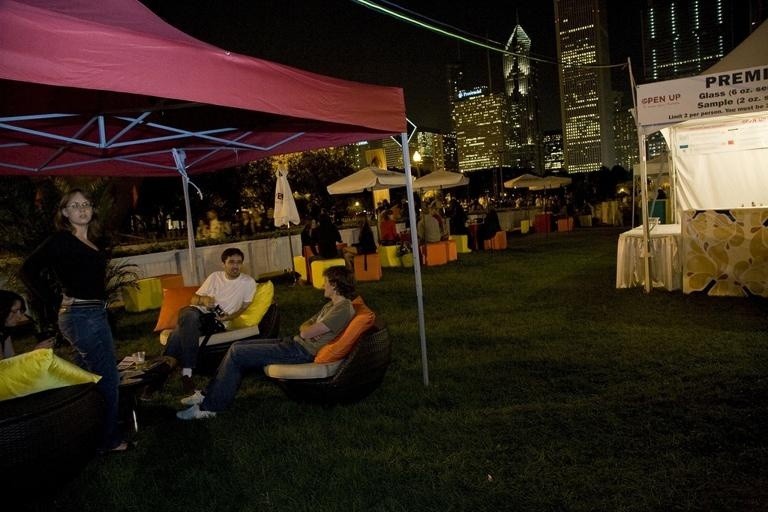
[176, 265, 357, 422]
[196, 208, 265, 242]
[142, 248, 257, 396]
[19, 187, 138, 454]
[0, 290, 54, 360]
[449, 189, 591, 251]
[299, 197, 468, 270]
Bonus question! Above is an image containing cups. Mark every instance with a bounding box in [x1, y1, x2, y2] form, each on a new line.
[133, 351, 146, 365]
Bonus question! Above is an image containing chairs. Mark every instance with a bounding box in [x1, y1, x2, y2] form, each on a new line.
[1, 225, 396, 433]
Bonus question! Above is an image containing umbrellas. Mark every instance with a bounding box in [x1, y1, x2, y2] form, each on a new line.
[273, 160, 301, 283]
[413, 170, 469, 216]
[326, 166, 416, 222]
[503, 173, 544, 208]
[528, 175, 572, 212]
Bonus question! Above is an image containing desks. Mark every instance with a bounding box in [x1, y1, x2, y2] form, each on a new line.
[615, 222, 684, 293]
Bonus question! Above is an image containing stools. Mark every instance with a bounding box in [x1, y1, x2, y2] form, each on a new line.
[287, 185, 640, 289]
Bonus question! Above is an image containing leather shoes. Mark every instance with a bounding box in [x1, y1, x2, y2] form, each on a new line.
[88, 438, 138, 456]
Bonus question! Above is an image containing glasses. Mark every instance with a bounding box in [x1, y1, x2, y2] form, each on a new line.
[60, 202, 93, 210]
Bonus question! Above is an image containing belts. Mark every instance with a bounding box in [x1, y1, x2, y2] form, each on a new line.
[64, 299, 106, 308]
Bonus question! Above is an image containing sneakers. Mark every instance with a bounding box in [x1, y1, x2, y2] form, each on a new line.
[174, 375, 223, 423]
[140, 380, 158, 407]
